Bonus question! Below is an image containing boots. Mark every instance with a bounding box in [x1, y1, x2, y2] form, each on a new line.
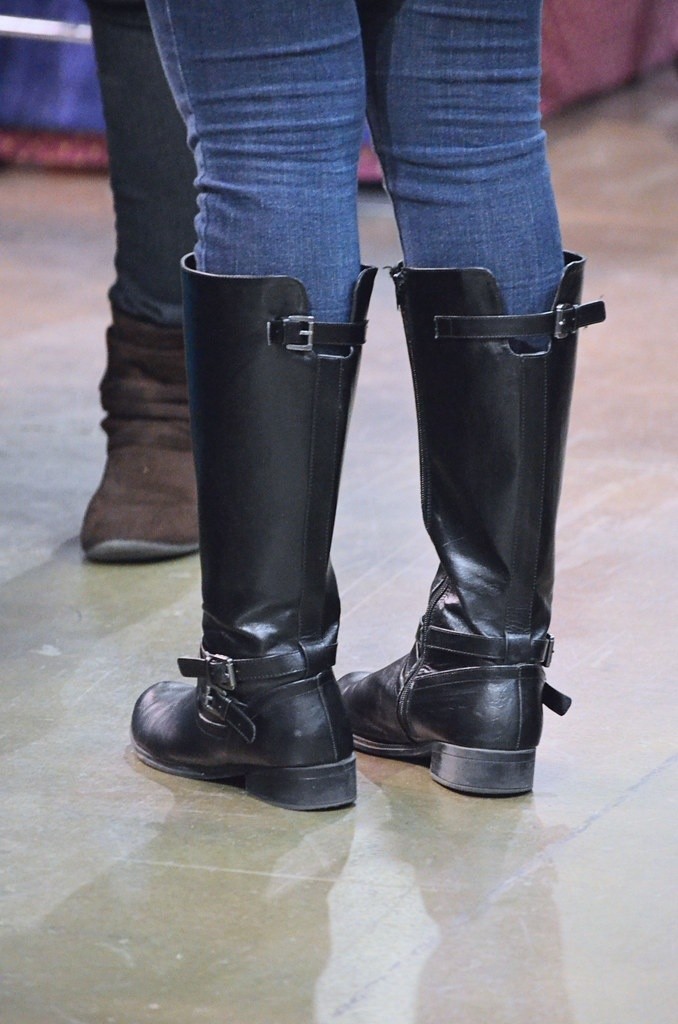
[81, 307, 198, 560]
[340, 252, 608, 796]
[129, 253, 377, 813]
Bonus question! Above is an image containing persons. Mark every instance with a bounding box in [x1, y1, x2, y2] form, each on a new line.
[130, 0, 607, 811]
[80, 0, 201, 562]
